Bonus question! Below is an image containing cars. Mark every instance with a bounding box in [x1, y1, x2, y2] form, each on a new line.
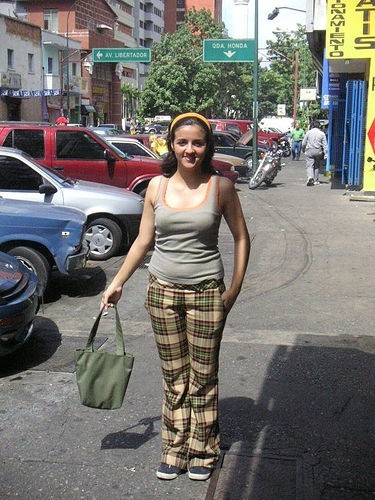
[0, 147, 147, 357]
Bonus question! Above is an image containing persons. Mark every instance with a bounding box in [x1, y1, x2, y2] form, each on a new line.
[56, 117, 69, 128]
[302, 121, 329, 186]
[290, 124, 305, 160]
[100, 113, 251, 481]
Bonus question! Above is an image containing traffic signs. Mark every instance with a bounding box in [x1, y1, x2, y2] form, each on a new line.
[204, 40, 257, 62]
[93, 47, 153, 63]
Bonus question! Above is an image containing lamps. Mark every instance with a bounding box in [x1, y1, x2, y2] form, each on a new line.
[267, 5, 305, 20]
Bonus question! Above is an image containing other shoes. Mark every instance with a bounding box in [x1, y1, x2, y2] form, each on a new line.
[313, 181, 320, 185]
[307, 177, 314, 185]
[156, 463, 182, 479]
[188, 466, 210, 480]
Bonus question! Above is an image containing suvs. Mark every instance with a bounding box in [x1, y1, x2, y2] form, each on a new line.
[0, 116, 289, 198]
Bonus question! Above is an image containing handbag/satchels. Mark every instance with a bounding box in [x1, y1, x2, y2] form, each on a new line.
[74, 302, 134, 410]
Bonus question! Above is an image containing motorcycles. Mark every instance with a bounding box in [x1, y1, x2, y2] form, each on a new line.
[249, 140, 284, 190]
